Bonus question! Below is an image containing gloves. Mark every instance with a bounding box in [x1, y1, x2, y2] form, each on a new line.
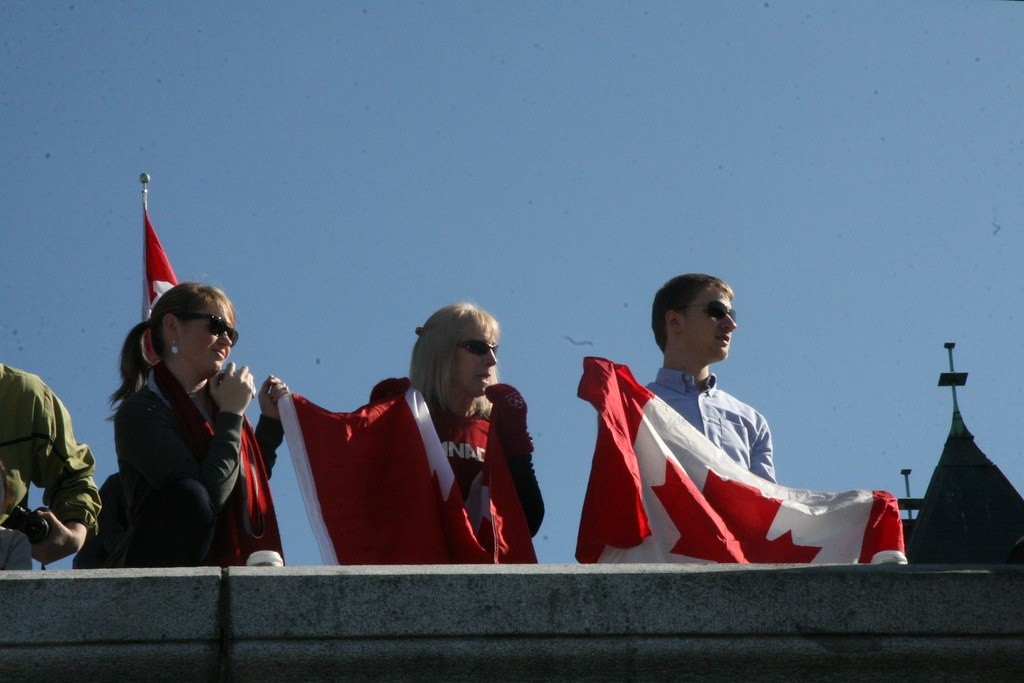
[369, 377, 411, 403]
[486, 384, 534, 456]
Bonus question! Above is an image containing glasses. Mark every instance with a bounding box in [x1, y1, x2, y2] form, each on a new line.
[178, 313, 239, 348]
[675, 301, 736, 323]
[456, 340, 498, 357]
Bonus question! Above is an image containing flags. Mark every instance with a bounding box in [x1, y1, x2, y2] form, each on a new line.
[268, 382, 540, 565]
[144, 207, 182, 303]
[577, 354, 911, 566]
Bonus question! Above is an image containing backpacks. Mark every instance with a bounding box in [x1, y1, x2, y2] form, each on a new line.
[72, 471, 133, 570]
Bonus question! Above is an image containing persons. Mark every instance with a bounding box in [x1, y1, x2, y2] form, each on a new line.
[104, 283, 287, 569]
[642, 270, 780, 482]
[0, 360, 102, 570]
[369, 303, 544, 538]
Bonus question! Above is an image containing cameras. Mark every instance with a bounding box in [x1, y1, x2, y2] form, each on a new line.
[220, 370, 256, 400]
[1, 504, 52, 543]
